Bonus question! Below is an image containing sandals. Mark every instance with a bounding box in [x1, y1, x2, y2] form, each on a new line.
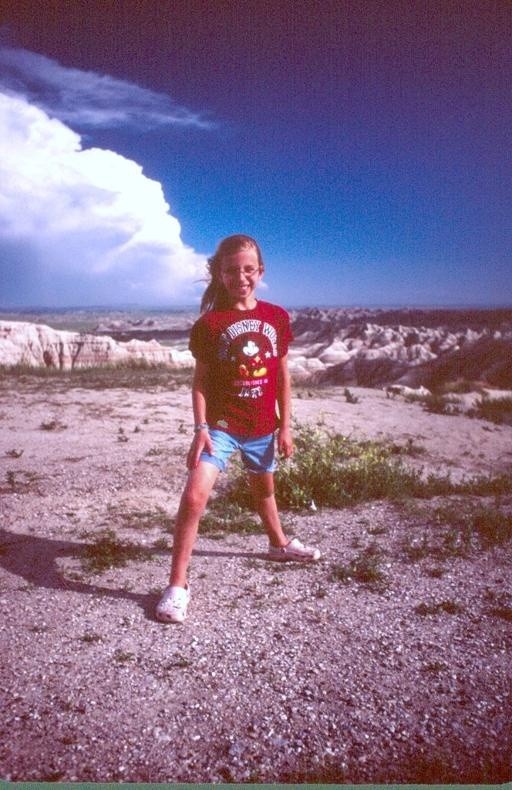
[268, 539, 321, 561]
[156, 583, 190, 623]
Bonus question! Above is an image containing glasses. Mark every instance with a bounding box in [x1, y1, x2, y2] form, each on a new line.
[220, 265, 260, 278]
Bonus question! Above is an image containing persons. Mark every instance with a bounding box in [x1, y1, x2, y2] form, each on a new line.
[153, 233, 323, 624]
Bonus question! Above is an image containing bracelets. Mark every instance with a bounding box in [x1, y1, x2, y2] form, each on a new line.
[193, 422, 209, 433]
